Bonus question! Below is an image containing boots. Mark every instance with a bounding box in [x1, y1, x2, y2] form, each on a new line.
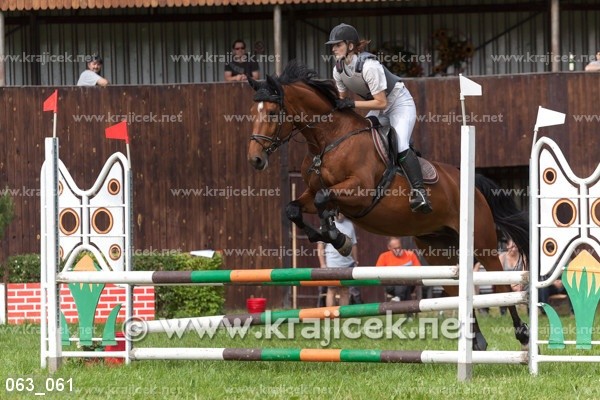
[399, 149, 433, 215]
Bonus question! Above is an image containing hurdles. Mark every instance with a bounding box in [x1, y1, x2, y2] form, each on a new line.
[40, 126, 600, 383]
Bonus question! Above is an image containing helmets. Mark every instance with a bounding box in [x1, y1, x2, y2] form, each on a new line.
[324, 23, 359, 45]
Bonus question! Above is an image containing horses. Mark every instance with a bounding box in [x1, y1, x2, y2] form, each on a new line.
[244, 59, 529, 350]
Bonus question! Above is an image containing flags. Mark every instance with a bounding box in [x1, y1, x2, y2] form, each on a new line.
[42, 88, 58, 114]
[534, 105, 567, 131]
[459, 73, 484, 99]
[104, 119, 128, 142]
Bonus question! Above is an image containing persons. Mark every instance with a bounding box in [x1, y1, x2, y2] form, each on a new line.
[223, 39, 260, 82]
[317, 209, 361, 310]
[324, 23, 431, 212]
[76, 56, 110, 86]
[498, 238, 531, 292]
[473, 263, 493, 311]
[584, 51, 600, 73]
[538, 245, 576, 316]
[375, 236, 424, 303]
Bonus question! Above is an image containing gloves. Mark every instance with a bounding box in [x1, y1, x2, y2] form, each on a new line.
[334, 97, 355, 112]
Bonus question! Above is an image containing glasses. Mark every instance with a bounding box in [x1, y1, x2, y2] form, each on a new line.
[234, 47, 246, 50]
